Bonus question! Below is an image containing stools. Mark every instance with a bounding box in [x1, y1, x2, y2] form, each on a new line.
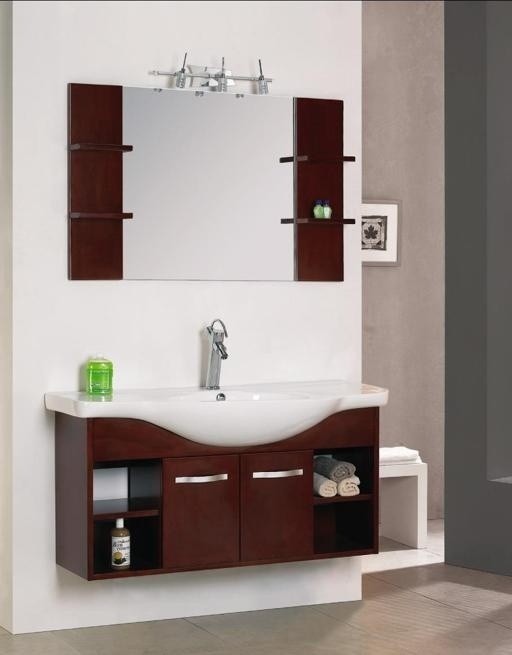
[380, 461, 429, 550]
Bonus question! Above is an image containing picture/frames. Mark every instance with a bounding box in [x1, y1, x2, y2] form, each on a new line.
[360, 196, 401, 268]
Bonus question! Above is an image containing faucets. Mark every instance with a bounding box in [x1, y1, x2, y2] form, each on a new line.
[204, 318, 228, 387]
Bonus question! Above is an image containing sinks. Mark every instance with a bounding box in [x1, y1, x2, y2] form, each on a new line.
[165, 391, 320, 448]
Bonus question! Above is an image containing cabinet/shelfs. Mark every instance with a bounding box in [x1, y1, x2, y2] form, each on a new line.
[69, 140, 134, 221]
[279, 156, 294, 225]
[54, 408, 162, 585]
[296, 154, 356, 225]
[313, 408, 381, 558]
[162, 452, 313, 576]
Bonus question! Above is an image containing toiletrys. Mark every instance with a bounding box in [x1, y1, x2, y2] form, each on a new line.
[111, 518, 131, 570]
[85, 353, 112, 394]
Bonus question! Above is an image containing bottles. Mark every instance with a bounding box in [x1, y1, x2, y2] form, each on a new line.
[109, 517, 133, 571]
[312, 198, 333, 219]
[86, 351, 114, 396]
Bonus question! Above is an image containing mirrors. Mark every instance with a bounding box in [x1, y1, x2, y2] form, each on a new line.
[124, 86, 295, 284]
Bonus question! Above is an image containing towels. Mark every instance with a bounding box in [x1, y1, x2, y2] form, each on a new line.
[314, 455, 362, 497]
[379, 446, 421, 464]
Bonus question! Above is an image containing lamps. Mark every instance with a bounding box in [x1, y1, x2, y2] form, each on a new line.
[156, 52, 273, 93]
